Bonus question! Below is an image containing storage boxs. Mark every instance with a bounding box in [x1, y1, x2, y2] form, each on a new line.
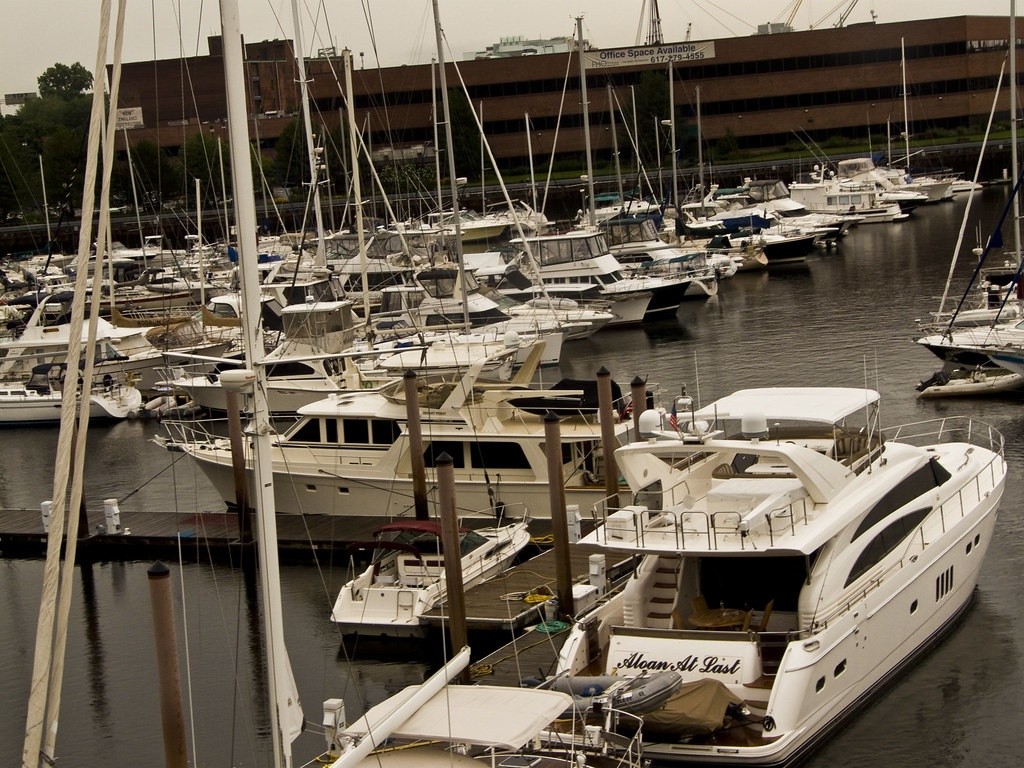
[571, 584, 599, 619]
[606, 505, 649, 544]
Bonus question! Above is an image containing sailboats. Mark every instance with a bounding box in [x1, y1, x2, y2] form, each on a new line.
[0, 1, 1024, 768]
